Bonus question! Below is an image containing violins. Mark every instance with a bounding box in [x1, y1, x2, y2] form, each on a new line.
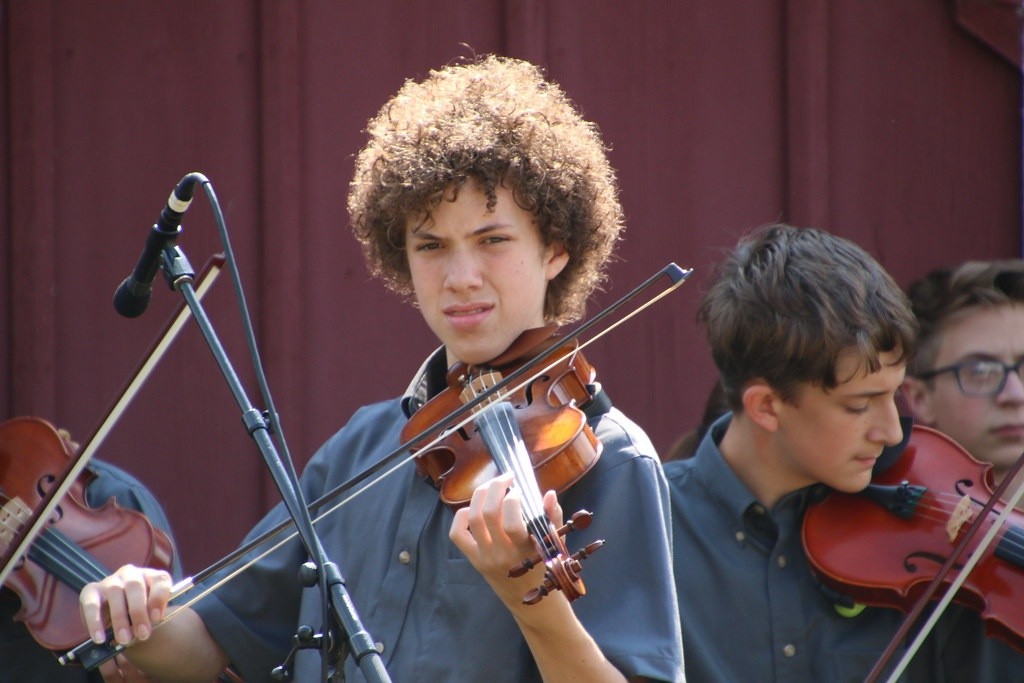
[793, 416, 1024, 661]
[0, 406, 253, 683]
[395, 310, 611, 610]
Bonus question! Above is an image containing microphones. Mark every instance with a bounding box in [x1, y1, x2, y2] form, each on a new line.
[113, 174, 198, 318]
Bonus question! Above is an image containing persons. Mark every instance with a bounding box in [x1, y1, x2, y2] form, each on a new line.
[662, 224, 933, 681]
[0, 418, 181, 683]
[78, 42, 683, 682]
[901, 260, 1024, 511]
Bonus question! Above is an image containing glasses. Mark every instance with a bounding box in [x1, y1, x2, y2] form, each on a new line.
[920, 356, 1024, 397]
